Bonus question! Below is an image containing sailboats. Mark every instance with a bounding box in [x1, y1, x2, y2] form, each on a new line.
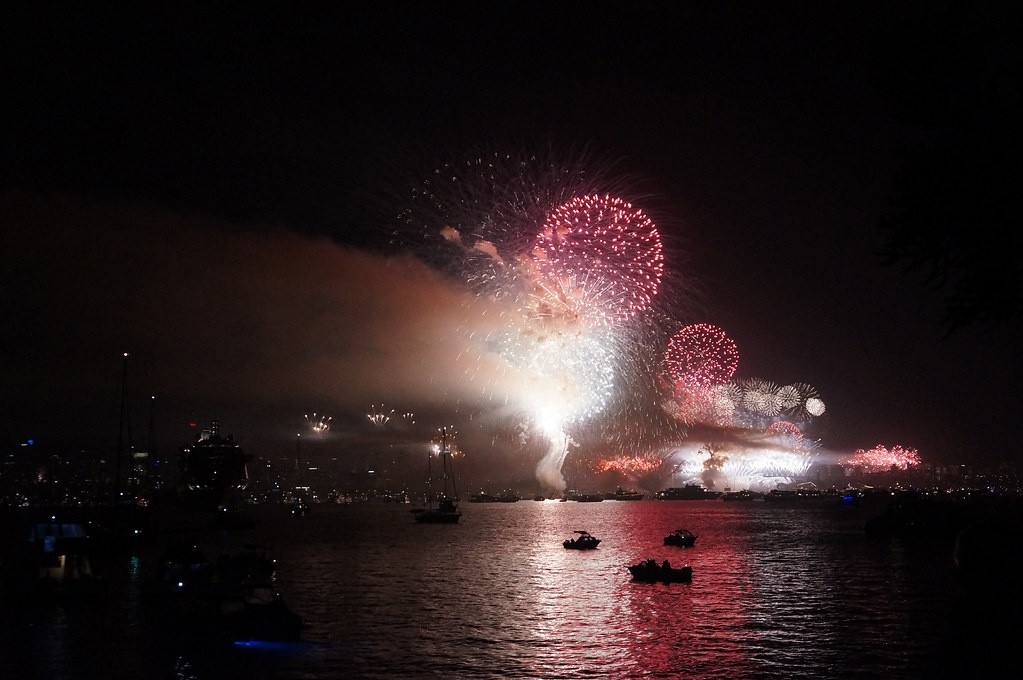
[409, 426, 464, 524]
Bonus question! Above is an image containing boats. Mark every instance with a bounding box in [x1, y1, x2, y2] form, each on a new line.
[625, 559, 693, 583]
[563, 530, 601, 549]
[472, 482, 944, 508]
[663, 529, 699, 546]
